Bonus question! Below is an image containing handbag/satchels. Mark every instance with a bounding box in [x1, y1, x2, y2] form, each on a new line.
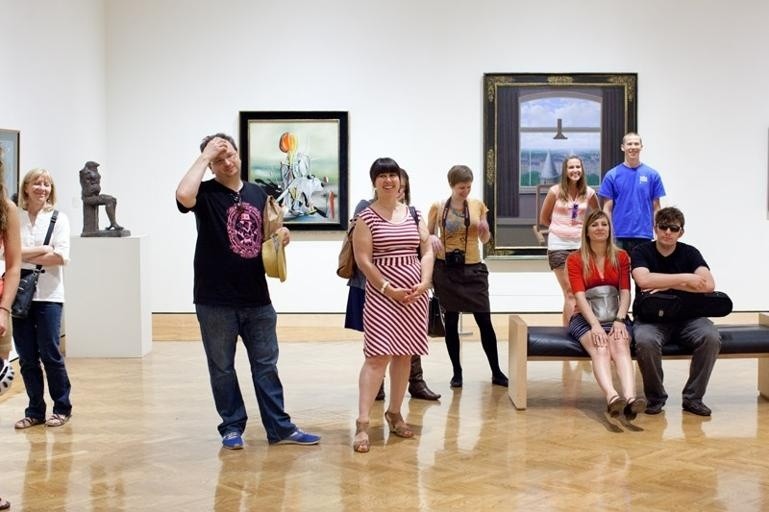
[0, 268, 41, 319]
[335, 234, 354, 279]
[427, 296, 445, 338]
[264, 194, 283, 237]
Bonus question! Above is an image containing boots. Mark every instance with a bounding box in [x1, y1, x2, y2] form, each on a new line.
[408, 354, 441, 401]
[374, 378, 385, 401]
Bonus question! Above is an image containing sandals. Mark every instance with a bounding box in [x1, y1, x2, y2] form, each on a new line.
[44, 414, 73, 427]
[14, 417, 47, 429]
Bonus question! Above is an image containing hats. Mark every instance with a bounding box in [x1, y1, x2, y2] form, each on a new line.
[261, 230, 287, 283]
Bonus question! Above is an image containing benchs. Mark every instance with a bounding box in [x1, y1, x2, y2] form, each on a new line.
[508, 313, 769, 410]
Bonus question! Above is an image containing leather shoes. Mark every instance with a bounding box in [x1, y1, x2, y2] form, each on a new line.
[682, 401, 712, 416]
[644, 400, 666, 414]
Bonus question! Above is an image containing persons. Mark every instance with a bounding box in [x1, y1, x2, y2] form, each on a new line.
[79, 160, 125, 231]
[345, 170, 442, 400]
[0, 153, 71, 511]
[426, 163, 508, 388]
[351, 156, 434, 453]
[599, 133, 664, 253]
[540, 157, 602, 328]
[631, 208, 721, 415]
[566, 209, 648, 421]
[178, 134, 320, 450]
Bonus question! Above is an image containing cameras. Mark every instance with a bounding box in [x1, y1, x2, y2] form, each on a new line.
[444, 250, 465, 269]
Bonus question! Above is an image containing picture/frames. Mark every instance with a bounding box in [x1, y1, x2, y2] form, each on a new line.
[239, 112, 348, 230]
[481, 72, 637, 260]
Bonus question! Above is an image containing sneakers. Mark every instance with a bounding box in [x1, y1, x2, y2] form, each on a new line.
[450, 377, 462, 387]
[492, 377, 508, 387]
[221, 432, 245, 450]
[274, 430, 321, 445]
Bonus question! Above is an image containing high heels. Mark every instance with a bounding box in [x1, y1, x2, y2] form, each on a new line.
[607, 395, 627, 418]
[623, 398, 647, 421]
[384, 410, 414, 438]
[353, 421, 370, 453]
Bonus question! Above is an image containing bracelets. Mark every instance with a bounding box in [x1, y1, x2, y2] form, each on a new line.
[613, 314, 630, 326]
[378, 280, 391, 295]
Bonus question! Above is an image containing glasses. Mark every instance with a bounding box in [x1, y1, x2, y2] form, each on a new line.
[573, 204, 578, 218]
[212, 152, 235, 165]
[657, 223, 680, 232]
